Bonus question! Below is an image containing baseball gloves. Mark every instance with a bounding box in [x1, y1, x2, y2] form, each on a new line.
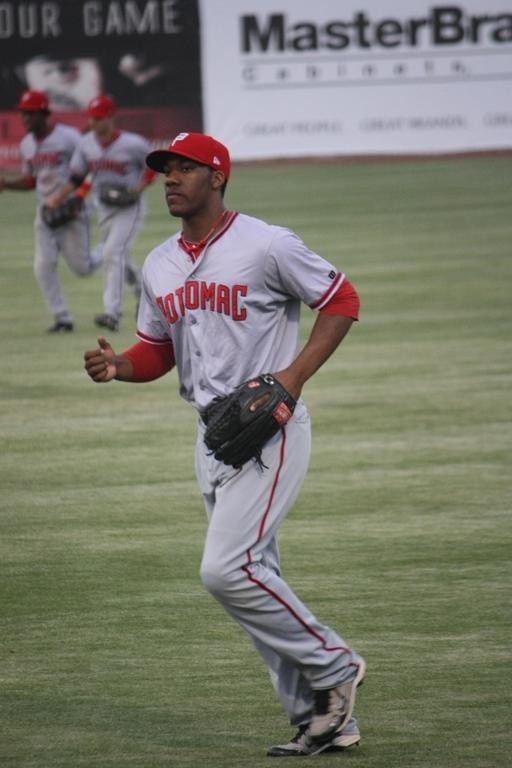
[41, 199, 80, 229]
[99, 182, 136, 207]
[200, 373, 295, 469]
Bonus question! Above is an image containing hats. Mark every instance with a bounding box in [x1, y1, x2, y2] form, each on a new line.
[146, 133, 230, 176]
[85, 94, 115, 119]
[15, 92, 49, 112]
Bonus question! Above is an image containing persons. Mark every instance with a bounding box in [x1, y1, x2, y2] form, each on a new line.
[83, 129, 368, 759]
[42, 93, 162, 333]
[17, 53, 177, 114]
[0, 90, 106, 335]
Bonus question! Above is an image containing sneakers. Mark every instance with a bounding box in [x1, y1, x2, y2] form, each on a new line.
[48, 323, 72, 333]
[97, 313, 120, 331]
[308, 655, 366, 744]
[266, 717, 361, 758]
